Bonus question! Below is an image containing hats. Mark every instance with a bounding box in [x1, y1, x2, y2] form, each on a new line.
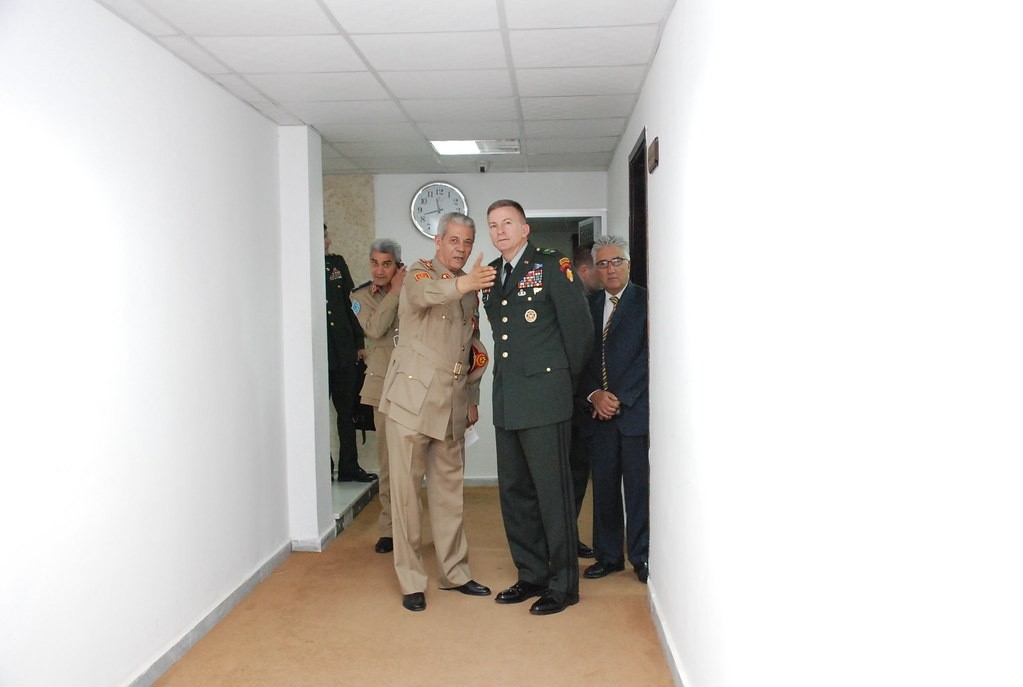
[468, 336, 489, 385]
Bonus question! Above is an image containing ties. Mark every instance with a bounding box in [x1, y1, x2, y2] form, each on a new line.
[502, 263, 513, 292]
[602, 296, 620, 391]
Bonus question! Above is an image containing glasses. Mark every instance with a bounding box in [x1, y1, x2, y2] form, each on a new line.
[596, 257, 629, 269]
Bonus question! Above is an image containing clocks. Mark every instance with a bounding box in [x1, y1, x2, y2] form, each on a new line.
[409, 180, 469, 239]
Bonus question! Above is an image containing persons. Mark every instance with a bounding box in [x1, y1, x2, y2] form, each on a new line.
[567, 240, 605, 558]
[377, 212, 497, 611]
[478, 199, 594, 615]
[349, 239, 409, 553]
[324, 224, 378, 483]
[580, 234, 650, 583]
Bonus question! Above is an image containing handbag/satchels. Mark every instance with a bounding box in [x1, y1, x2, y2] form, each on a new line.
[355, 404, 376, 445]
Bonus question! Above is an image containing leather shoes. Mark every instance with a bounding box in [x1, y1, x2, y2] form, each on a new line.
[494, 582, 548, 604]
[338, 467, 378, 482]
[403, 593, 426, 610]
[440, 579, 491, 596]
[633, 561, 648, 584]
[375, 537, 393, 553]
[530, 589, 579, 615]
[582, 562, 624, 578]
[578, 540, 594, 558]
